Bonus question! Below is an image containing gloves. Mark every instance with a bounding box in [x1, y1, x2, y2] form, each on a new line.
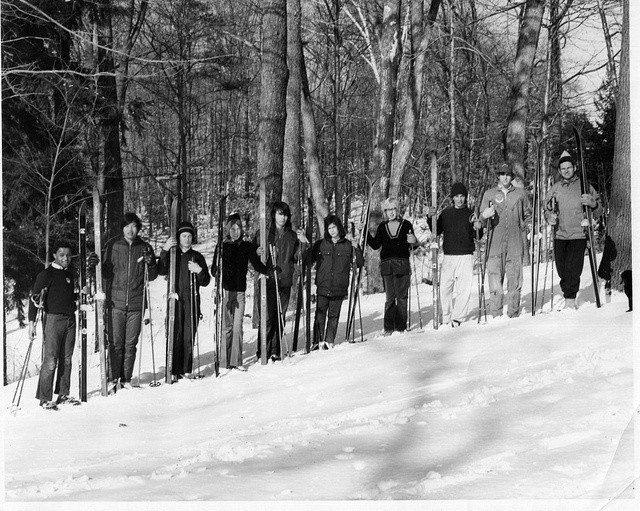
[143, 246, 156, 267]
[473, 220, 482, 230]
[296, 226, 307, 242]
[88, 253, 99, 266]
[407, 234, 416, 243]
[266, 265, 282, 279]
[211, 265, 225, 277]
[428, 206, 437, 218]
[482, 205, 495, 219]
[359, 221, 364, 233]
[544, 211, 557, 225]
[256, 247, 264, 257]
[580, 194, 596, 208]
[352, 238, 360, 248]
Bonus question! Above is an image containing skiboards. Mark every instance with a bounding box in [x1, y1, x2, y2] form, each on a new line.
[259, 175, 270, 367]
[92, 187, 108, 398]
[78, 200, 88, 403]
[292, 197, 314, 355]
[214, 191, 231, 378]
[430, 149, 439, 331]
[344, 174, 383, 341]
[527, 135, 549, 318]
[164, 192, 183, 386]
[568, 119, 602, 308]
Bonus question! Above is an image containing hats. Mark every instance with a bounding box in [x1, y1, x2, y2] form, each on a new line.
[226, 213, 244, 239]
[495, 161, 517, 181]
[176, 221, 195, 243]
[558, 150, 576, 168]
[451, 182, 468, 198]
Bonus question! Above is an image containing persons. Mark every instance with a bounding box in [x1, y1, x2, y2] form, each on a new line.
[478, 162, 533, 319]
[28, 241, 81, 411]
[157, 221, 211, 385]
[88, 213, 158, 396]
[211, 219, 276, 372]
[304, 215, 364, 351]
[252, 202, 307, 363]
[358, 198, 419, 338]
[427, 183, 483, 327]
[543, 156, 603, 310]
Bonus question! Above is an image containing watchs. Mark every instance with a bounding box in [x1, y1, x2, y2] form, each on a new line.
[591, 203, 599, 211]
